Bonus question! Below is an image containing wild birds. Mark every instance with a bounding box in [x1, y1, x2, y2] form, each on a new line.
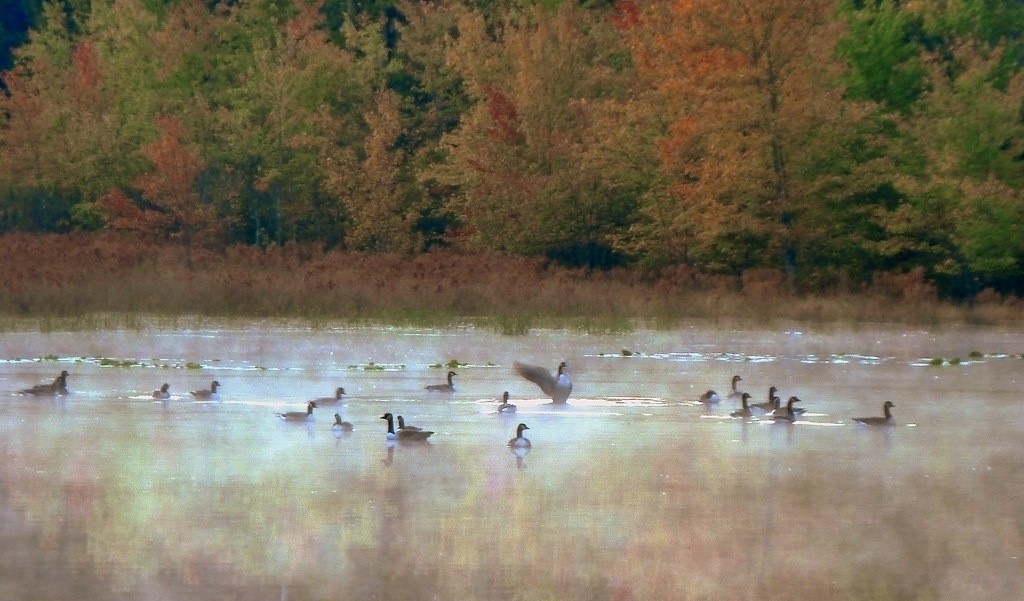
[151, 383, 171, 399]
[281, 401, 317, 422]
[25, 370, 70, 396]
[312, 386, 346, 405]
[498, 390, 516, 412]
[515, 361, 573, 404]
[190, 381, 221, 399]
[332, 413, 353, 430]
[424, 371, 458, 393]
[852, 401, 896, 426]
[509, 423, 531, 447]
[698, 375, 807, 422]
[380, 413, 434, 441]
